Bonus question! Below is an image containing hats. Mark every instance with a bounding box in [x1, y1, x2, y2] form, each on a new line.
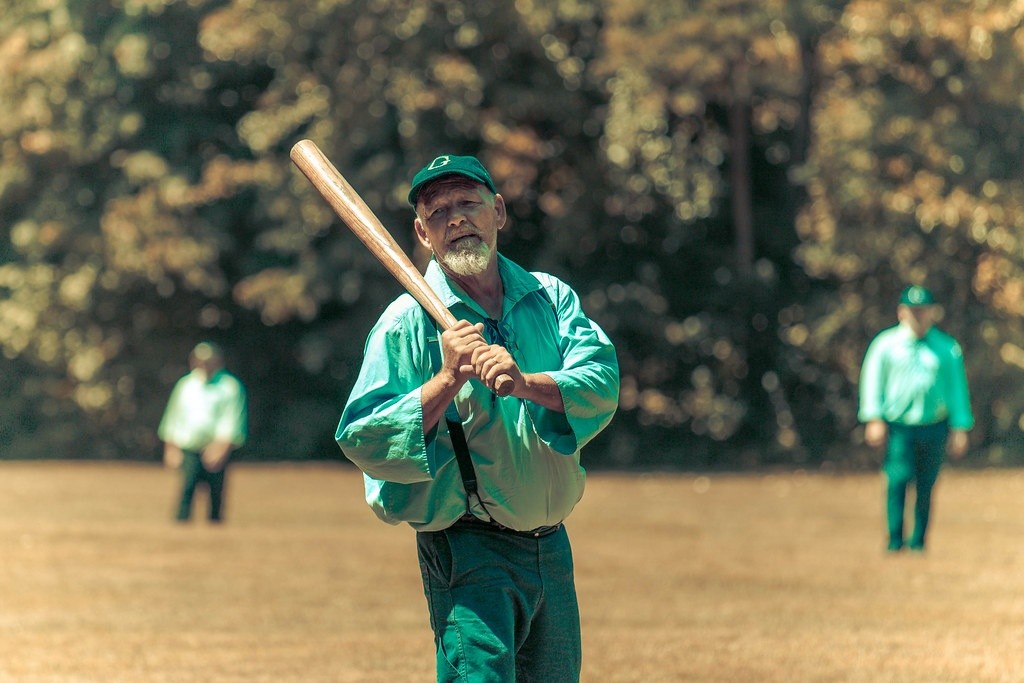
[407, 152, 497, 213]
[895, 286, 936, 309]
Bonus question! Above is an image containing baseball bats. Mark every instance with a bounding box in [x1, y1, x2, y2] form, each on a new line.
[289, 137, 517, 397]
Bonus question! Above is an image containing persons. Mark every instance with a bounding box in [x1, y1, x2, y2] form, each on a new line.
[157, 341, 247, 521]
[856, 284, 975, 551]
[335, 155, 619, 683]
